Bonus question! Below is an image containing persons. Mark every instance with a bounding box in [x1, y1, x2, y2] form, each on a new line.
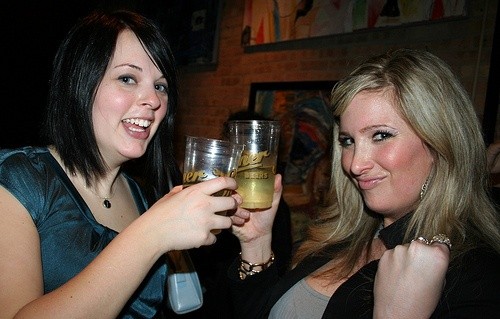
[228, 48, 500, 319]
[0, 10, 237, 319]
[202, 110, 292, 319]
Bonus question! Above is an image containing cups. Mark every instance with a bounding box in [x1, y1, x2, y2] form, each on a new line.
[227, 120, 281, 210]
[183, 136, 247, 234]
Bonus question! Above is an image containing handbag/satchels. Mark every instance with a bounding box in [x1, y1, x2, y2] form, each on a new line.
[126, 174, 203, 313]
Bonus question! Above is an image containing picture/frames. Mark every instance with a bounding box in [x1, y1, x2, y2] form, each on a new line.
[249, 78, 335, 260]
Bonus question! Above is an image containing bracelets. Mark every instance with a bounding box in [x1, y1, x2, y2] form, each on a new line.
[237, 250, 274, 280]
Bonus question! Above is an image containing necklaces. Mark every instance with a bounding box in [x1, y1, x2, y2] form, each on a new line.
[76, 176, 120, 208]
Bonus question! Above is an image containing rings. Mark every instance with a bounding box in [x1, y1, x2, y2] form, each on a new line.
[430, 234, 452, 249]
[411, 236, 429, 244]
